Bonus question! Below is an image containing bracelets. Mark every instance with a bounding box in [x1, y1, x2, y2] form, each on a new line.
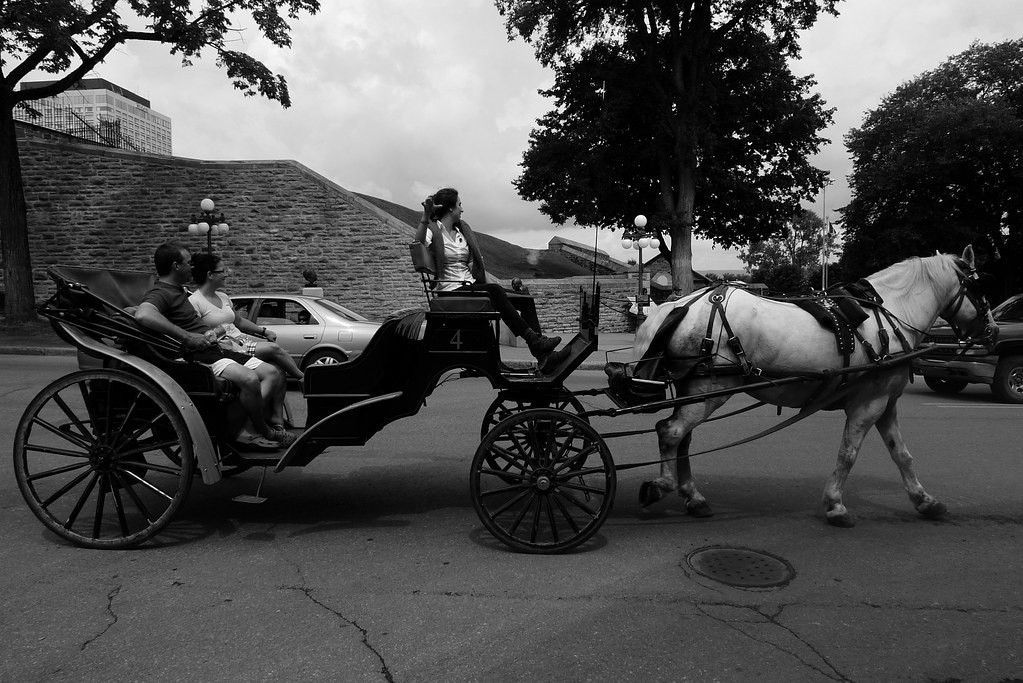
[262, 327, 266, 336]
[420, 220, 429, 225]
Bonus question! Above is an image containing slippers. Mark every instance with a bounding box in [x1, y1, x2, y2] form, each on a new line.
[235, 430, 281, 448]
[263, 418, 286, 433]
[268, 427, 296, 447]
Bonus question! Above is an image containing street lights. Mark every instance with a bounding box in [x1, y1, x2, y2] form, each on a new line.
[188, 198, 229, 254]
[621, 215, 660, 328]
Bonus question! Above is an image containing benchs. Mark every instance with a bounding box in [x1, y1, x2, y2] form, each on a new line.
[114, 303, 242, 404]
[409, 241, 534, 317]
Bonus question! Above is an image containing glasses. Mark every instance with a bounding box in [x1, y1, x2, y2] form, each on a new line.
[298, 318, 307, 322]
[211, 267, 228, 276]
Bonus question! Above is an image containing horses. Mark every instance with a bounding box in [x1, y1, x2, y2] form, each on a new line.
[632, 244, 1000, 526]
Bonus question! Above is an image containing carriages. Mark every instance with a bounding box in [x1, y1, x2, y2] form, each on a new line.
[12, 242, 1002, 557]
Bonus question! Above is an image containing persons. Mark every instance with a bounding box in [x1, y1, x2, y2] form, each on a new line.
[409, 188, 562, 362]
[135, 242, 304, 449]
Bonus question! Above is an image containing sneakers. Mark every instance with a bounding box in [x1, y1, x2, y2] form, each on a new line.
[525, 333, 562, 356]
[538, 344, 572, 376]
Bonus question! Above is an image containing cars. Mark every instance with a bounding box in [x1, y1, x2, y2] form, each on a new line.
[913, 294, 1023, 405]
[228, 294, 426, 374]
